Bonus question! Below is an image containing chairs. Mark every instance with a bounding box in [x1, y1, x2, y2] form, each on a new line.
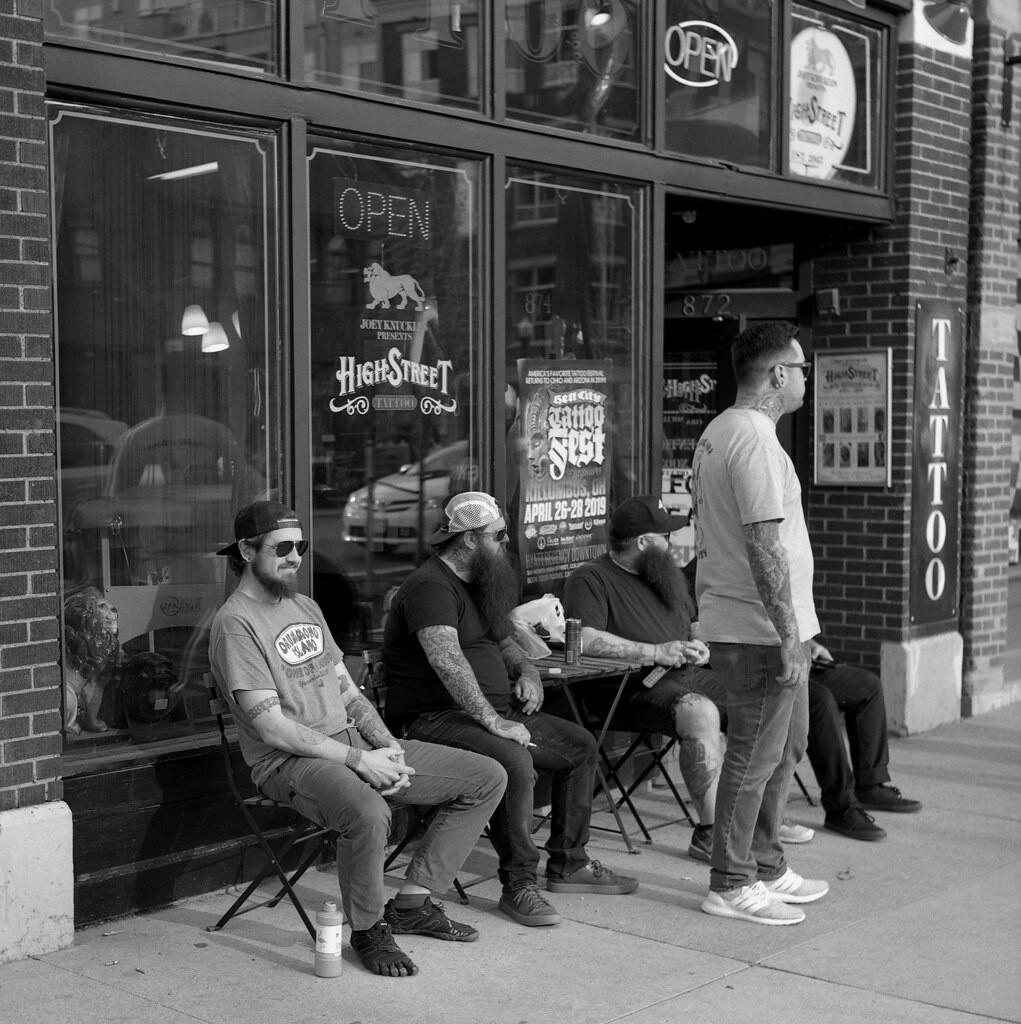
[204, 646, 817, 945]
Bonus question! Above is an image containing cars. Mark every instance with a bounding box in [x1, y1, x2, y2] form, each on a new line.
[342, 439, 470, 559]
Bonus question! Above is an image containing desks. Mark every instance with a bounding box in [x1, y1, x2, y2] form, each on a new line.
[507, 648, 655, 856]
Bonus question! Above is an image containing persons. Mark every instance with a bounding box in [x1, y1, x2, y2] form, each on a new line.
[382, 491, 641, 929]
[105, 353, 241, 577]
[690, 320, 831, 927]
[207, 499, 509, 979]
[562, 494, 817, 869]
[808, 638, 922, 843]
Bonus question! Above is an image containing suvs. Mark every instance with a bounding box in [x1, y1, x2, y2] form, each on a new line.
[54, 406, 164, 551]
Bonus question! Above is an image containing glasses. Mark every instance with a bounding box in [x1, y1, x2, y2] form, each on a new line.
[262, 540, 308, 557]
[471, 525, 507, 542]
[768, 362, 811, 378]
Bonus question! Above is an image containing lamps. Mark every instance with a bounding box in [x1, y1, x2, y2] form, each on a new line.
[923, 0, 974, 45]
[182, 305, 230, 353]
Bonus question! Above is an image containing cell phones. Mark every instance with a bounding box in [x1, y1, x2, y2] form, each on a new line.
[814, 657, 835, 668]
[642, 665, 673, 689]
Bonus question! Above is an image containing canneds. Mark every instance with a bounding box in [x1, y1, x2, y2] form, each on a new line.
[564, 618, 582, 665]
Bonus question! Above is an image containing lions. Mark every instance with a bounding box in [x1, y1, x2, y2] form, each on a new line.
[64, 585, 121, 737]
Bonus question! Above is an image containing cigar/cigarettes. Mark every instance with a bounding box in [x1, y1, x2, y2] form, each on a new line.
[524, 742, 537, 748]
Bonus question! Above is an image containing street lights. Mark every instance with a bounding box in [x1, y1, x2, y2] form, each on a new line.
[517, 316, 534, 359]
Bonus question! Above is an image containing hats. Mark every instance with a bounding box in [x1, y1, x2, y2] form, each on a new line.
[608, 495, 688, 540]
[425, 491, 504, 545]
[216, 501, 303, 555]
[66, 495, 140, 551]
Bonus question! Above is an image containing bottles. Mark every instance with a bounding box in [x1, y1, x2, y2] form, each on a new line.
[315, 901, 344, 978]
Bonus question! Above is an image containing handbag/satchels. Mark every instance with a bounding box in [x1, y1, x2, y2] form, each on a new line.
[508, 615, 552, 660]
[507, 593, 565, 643]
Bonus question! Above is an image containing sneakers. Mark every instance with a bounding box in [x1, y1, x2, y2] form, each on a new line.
[350, 919, 419, 977]
[701, 880, 805, 926]
[762, 866, 829, 903]
[499, 884, 563, 927]
[688, 823, 715, 866]
[547, 859, 638, 894]
[824, 805, 887, 841]
[384, 896, 479, 942]
[779, 817, 815, 843]
[854, 784, 922, 816]
